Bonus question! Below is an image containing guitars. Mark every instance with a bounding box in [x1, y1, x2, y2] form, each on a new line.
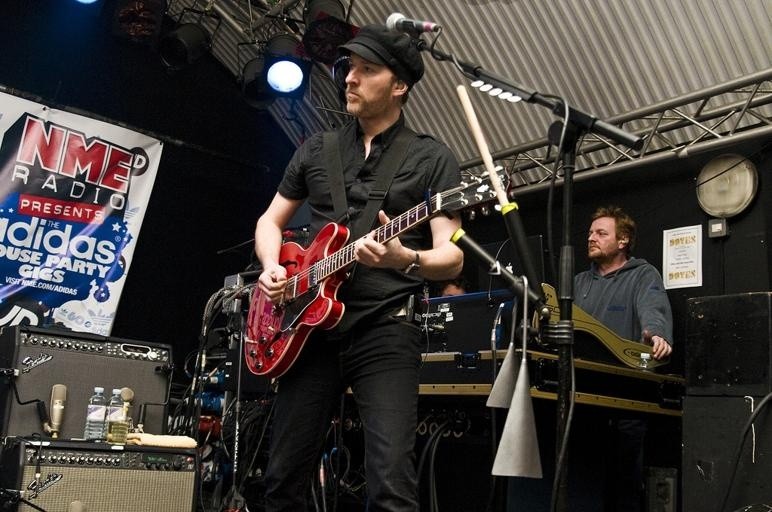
[243, 166, 516, 379]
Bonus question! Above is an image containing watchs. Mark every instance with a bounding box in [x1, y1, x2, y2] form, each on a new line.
[398, 255, 421, 277]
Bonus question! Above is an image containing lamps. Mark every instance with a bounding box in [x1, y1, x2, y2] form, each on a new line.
[160, 0, 351, 108]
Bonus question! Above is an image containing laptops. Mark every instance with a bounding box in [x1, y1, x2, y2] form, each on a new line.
[478, 234, 545, 291]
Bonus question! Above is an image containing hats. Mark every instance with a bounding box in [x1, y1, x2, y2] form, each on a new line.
[338, 23, 425, 83]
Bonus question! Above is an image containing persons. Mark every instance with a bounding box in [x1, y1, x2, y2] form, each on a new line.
[253, 21, 465, 511]
[432, 279, 474, 299]
[572, 206, 675, 511]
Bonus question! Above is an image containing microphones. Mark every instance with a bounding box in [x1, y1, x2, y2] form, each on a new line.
[386, 12, 440, 36]
[69, 501, 83, 512]
[50, 383, 67, 438]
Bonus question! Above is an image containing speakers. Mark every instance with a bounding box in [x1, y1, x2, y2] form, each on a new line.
[5, 437, 200, 512]
[684, 293, 772, 397]
[1, 325, 174, 442]
[681, 396, 772, 512]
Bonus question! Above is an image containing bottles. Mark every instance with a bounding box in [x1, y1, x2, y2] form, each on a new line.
[640, 353, 651, 368]
[83, 386, 126, 440]
